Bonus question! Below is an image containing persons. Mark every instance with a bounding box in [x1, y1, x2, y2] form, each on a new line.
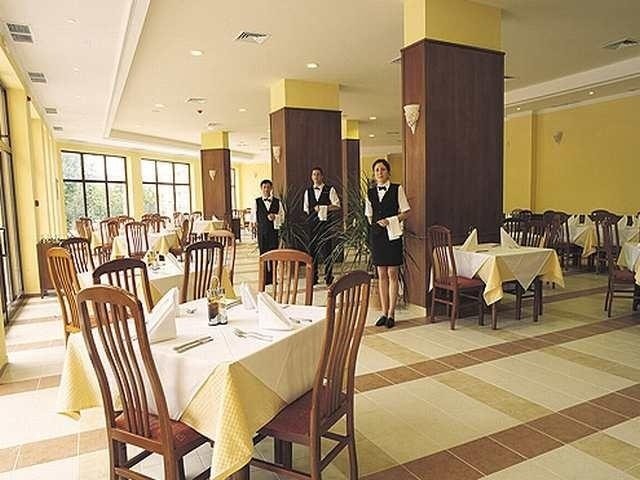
[364, 158, 412, 328]
[303, 168, 340, 286]
[249, 180, 285, 285]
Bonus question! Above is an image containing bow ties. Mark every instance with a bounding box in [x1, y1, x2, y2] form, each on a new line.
[314, 187, 321, 190]
[264, 198, 273, 202]
[378, 186, 387, 191]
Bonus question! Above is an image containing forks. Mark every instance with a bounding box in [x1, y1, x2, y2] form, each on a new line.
[235, 332, 271, 341]
[235, 327, 274, 338]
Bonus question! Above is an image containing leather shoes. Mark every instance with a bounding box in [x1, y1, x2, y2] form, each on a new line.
[387, 317, 394, 328]
[375, 316, 387, 326]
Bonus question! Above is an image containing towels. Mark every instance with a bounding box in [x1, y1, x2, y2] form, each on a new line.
[571, 214, 580, 225]
[165, 252, 183, 274]
[617, 215, 627, 227]
[239, 282, 257, 310]
[153, 287, 181, 317]
[318, 205, 328, 221]
[584, 214, 592, 224]
[385, 216, 403, 241]
[500, 226, 519, 248]
[212, 215, 218, 221]
[274, 214, 280, 230]
[568, 214, 574, 223]
[148, 304, 177, 342]
[461, 228, 478, 250]
[257, 291, 295, 331]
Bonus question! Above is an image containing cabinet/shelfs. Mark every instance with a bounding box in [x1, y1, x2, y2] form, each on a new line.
[36, 238, 66, 299]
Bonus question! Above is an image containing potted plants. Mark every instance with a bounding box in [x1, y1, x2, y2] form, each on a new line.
[308, 165, 424, 311]
[247, 182, 312, 278]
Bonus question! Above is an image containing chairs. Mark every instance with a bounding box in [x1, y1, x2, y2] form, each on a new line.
[559, 212, 584, 271]
[138, 211, 169, 231]
[170, 219, 190, 263]
[258, 249, 313, 307]
[208, 231, 237, 287]
[92, 258, 154, 319]
[119, 215, 136, 224]
[551, 223, 559, 242]
[502, 222, 551, 320]
[251, 269, 371, 480]
[426, 224, 484, 330]
[233, 208, 251, 227]
[499, 218, 532, 246]
[520, 210, 534, 219]
[59, 237, 95, 273]
[181, 241, 224, 302]
[108, 219, 119, 237]
[596, 212, 622, 271]
[592, 208, 608, 221]
[75, 284, 212, 480]
[172, 210, 202, 223]
[601, 218, 635, 318]
[45, 246, 121, 349]
[512, 208, 522, 216]
[80, 216, 94, 232]
[533, 207, 560, 222]
[125, 221, 149, 258]
[100, 221, 113, 264]
